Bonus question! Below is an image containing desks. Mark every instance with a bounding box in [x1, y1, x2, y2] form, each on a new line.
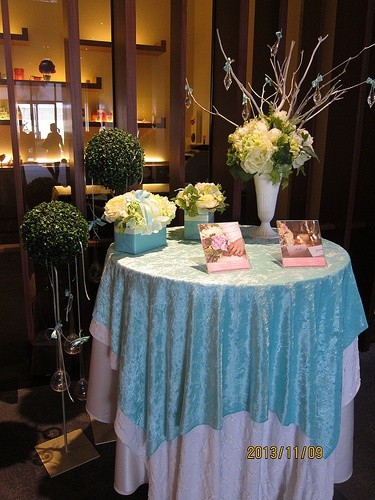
[86, 224, 368, 500]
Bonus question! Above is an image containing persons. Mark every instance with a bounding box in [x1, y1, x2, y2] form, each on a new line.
[42, 123, 64, 181]
[20, 123, 33, 162]
[218, 222, 245, 257]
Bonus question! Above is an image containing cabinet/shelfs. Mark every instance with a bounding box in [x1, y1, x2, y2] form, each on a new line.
[0, 0, 173, 171]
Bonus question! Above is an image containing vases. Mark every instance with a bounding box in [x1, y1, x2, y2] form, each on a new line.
[114, 225, 166, 255]
[183, 208, 214, 240]
[247, 170, 282, 239]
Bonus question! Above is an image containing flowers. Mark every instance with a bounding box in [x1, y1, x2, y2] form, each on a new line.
[103, 189, 177, 235]
[226, 107, 315, 190]
[171, 182, 229, 217]
